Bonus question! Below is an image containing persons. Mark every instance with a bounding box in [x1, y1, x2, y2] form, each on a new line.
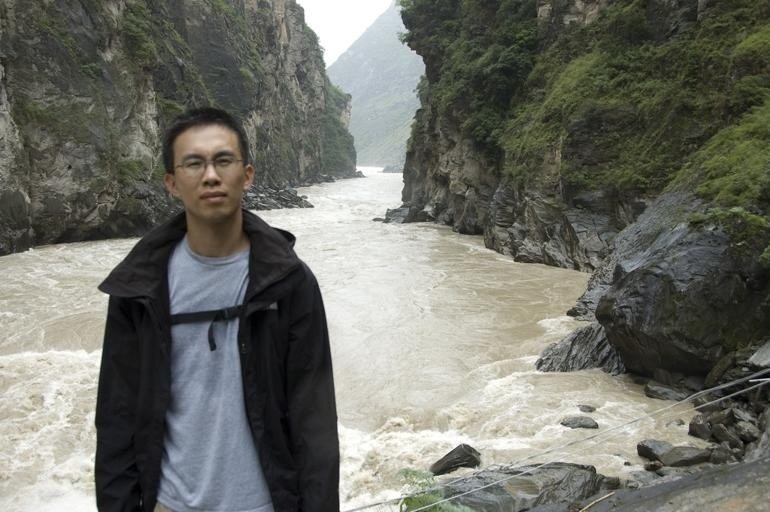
[94, 108, 340, 511]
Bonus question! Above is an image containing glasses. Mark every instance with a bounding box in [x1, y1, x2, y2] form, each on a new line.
[174, 156, 241, 177]
